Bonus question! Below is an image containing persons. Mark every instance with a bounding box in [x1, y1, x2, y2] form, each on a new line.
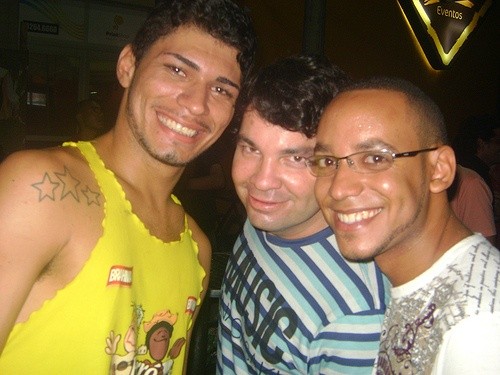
[305, 77, 500, 375]
[0, 1, 260, 374]
[446, 115, 500, 249]
[216, 51, 391, 375]
[65, 96, 106, 144]
[173, 153, 243, 374]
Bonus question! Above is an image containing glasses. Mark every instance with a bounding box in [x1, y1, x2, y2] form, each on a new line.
[304, 146, 440, 177]
